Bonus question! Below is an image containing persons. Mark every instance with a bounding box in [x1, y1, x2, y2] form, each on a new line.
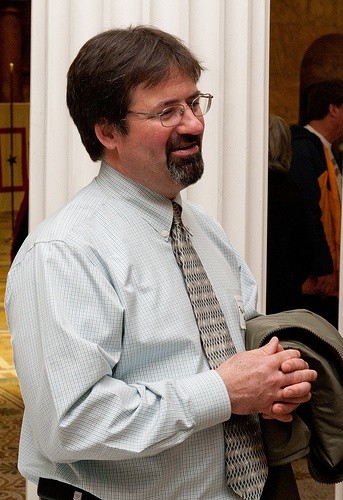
[267, 114, 291, 316]
[291, 78, 342, 328]
[4, 27, 318, 499]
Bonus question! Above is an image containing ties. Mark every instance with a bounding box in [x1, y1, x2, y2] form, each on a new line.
[169, 199, 269, 500]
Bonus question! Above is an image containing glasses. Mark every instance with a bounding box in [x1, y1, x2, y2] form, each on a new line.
[121, 94, 214, 128]
